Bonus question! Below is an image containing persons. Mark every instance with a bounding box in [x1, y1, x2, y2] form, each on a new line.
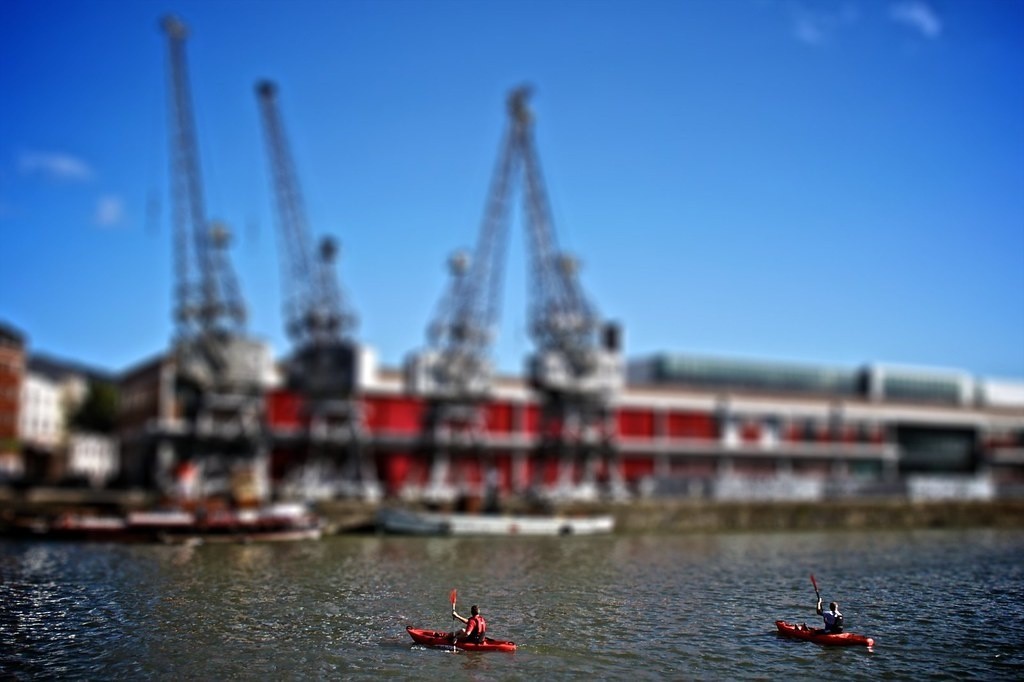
[446, 604, 486, 643]
[794, 597, 844, 634]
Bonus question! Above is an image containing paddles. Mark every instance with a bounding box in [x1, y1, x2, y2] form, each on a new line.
[450, 589, 456, 652]
[811, 573, 823, 612]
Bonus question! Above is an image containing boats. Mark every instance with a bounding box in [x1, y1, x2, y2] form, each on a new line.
[380, 495, 617, 539]
[774, 620, 875, 649]
[406, 624, 517, 654]
[11, 491, 324, 544]
